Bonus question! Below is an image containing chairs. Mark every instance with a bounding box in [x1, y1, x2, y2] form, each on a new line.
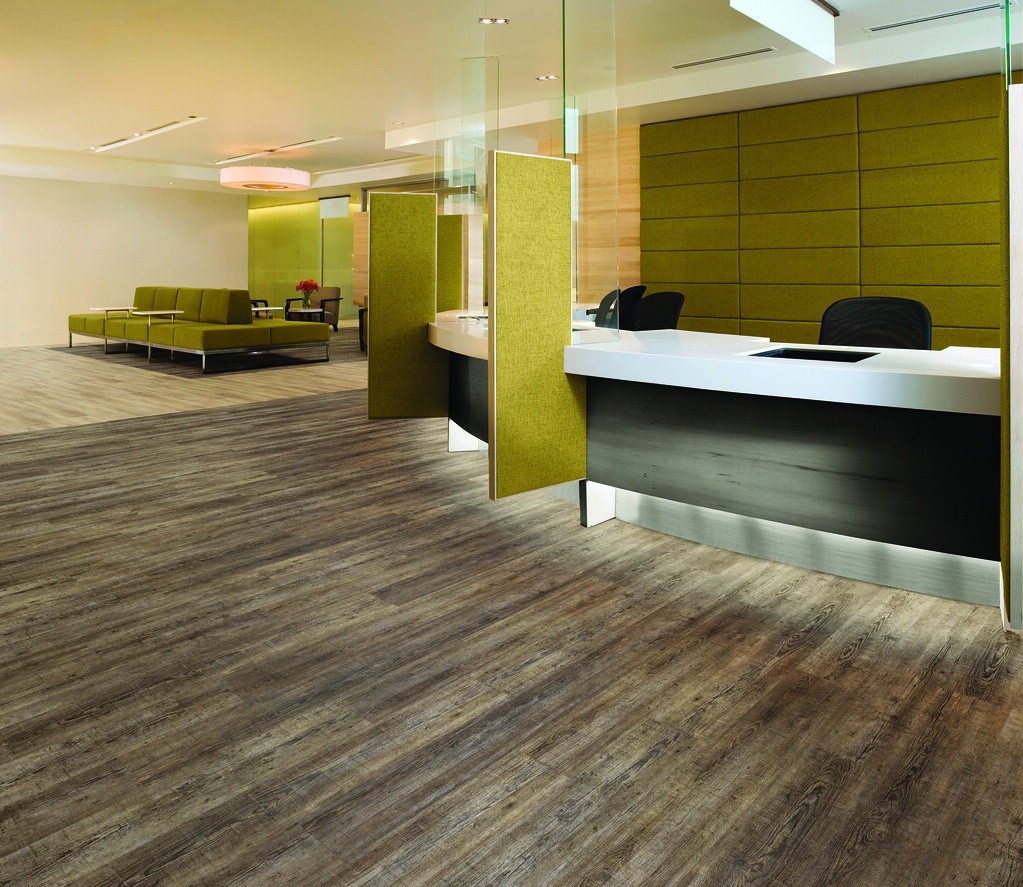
[594, 284, 685, 332]
[818, 296, 933, 350]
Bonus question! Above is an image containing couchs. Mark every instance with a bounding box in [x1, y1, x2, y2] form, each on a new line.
[284, 286, 343, 332]
[68, 287, 331, 373]
[359, 295, 368, 355]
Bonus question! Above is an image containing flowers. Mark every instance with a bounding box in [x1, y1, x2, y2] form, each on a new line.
[296, 279, 320, 306]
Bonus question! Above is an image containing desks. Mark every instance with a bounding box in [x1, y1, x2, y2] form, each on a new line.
[90, 307, 137, 321]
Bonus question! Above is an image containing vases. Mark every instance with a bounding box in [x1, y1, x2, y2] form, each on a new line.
[302, 296, 310, 309]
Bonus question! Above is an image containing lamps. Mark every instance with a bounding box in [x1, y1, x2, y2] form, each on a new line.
[220, 161, 311, 192]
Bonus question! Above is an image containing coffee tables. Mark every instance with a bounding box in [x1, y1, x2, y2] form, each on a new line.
[252, 306, 284, 319]
[131, 309, 183, 325]
[288, 307, 323, 322]
